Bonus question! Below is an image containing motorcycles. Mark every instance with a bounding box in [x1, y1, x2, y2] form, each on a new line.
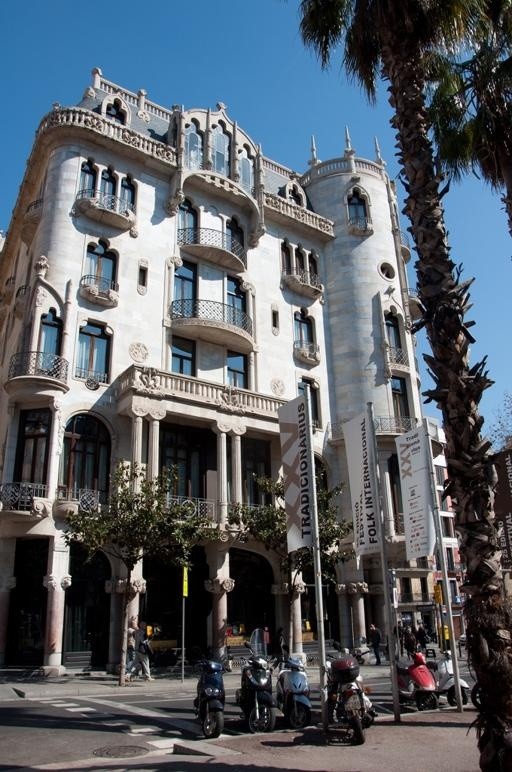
[392, 649, 482, 713]
[322, 645, 382, 747]
[192, 650, 233, 737]
[271, 644, 312, 728]
[237, 629, 281, 734]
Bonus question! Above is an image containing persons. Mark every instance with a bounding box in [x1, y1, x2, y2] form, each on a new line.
[126, 627, 137, 682]
[272, 627, 289, 669]
[123, 621, 155, 683]
[394, 619, 437, 661]
[367, 623, 381, 666]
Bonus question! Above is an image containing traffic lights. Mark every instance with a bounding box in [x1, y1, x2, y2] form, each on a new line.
[434, 584, 442, 603]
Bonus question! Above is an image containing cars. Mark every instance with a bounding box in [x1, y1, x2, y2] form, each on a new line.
[458, 631, 469, 645]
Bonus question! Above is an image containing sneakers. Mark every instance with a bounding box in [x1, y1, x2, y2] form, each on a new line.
[125, 674, 156, 683]
